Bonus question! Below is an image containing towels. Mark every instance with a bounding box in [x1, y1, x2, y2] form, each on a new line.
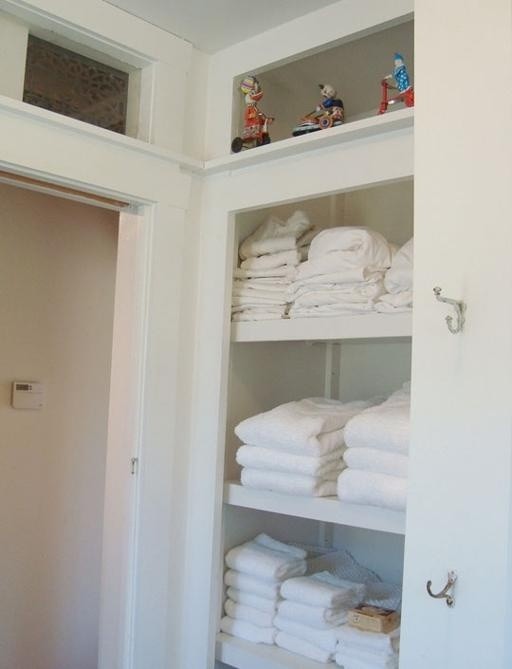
[220, 531, 403, 668]
[233, 379, 413, 514]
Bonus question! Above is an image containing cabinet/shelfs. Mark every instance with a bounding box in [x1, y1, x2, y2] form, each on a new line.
[205, 177, 417, 669]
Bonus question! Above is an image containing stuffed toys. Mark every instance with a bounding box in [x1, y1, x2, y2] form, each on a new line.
[291, 83, 347, 137]
[232, 74, 275, 153]
[378, 52, 414, 116]
